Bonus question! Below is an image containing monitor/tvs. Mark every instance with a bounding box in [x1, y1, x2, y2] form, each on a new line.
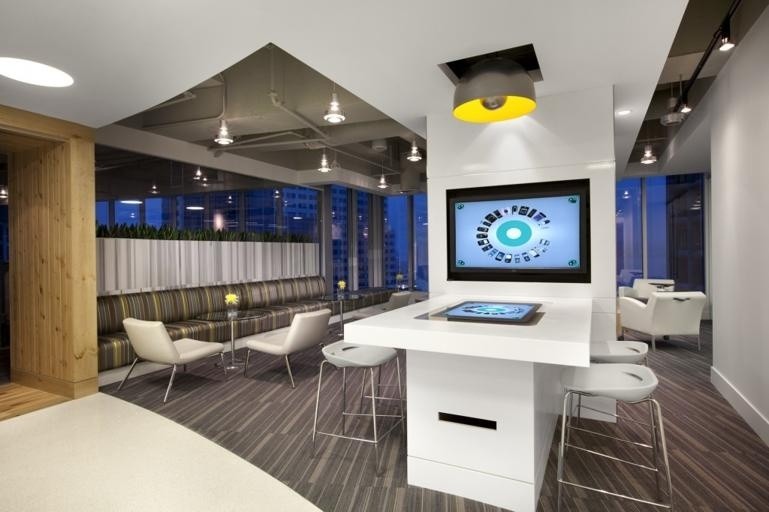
[432, 298, 541, 325]
[444, 179, 591, 285]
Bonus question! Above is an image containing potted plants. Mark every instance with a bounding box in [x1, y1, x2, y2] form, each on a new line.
[97, 221, 320, 297]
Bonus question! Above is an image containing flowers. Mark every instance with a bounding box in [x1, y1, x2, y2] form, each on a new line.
[336, 280, 346, 287]
[224, 292, 240, 305]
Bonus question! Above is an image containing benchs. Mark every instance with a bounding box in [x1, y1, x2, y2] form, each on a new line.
[96, 275, 389, 374]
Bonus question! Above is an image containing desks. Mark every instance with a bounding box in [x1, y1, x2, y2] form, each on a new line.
[344, 290, 595, 511]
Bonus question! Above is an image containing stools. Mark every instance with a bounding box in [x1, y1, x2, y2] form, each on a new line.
[559, 335, 674, 511]
[312, 339, 405, 474]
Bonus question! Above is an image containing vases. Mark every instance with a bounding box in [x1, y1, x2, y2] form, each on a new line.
[337, 287, 345, 298]
[227, 304, 238, 320]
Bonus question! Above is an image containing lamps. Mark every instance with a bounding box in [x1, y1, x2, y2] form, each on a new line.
[639, 35, 736, 166]
[452, 53, 536, 123]
[214, 71, 424, 190]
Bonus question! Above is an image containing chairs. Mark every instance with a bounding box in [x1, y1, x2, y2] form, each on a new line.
[620, 290, 706, 353]
[244, 308, 332, 388]
[389, 291, 412, 307]
[117, 317, 226, 403]
[618, 277, 675, 298]
